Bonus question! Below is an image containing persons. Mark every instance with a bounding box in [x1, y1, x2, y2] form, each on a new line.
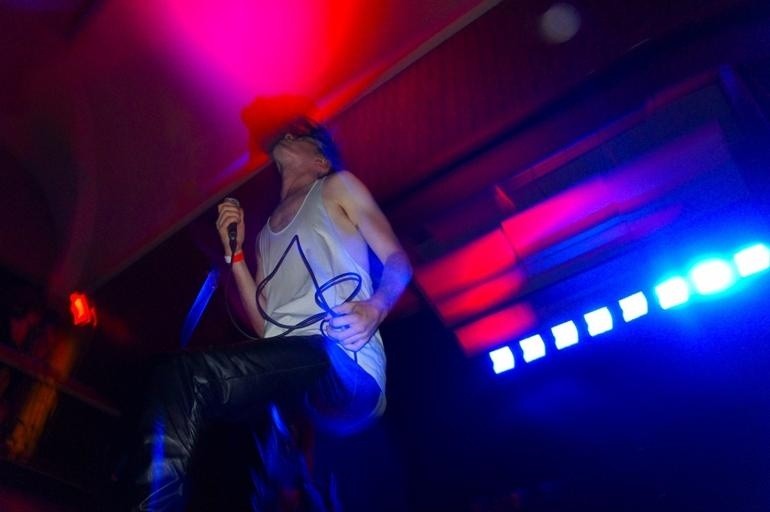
[124, 114, 418, 509]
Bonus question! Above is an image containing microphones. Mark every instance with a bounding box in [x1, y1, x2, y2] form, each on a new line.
[224, 197, 241, 252]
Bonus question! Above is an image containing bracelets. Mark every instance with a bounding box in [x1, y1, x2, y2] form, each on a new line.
[225, 252, 246, 264]
